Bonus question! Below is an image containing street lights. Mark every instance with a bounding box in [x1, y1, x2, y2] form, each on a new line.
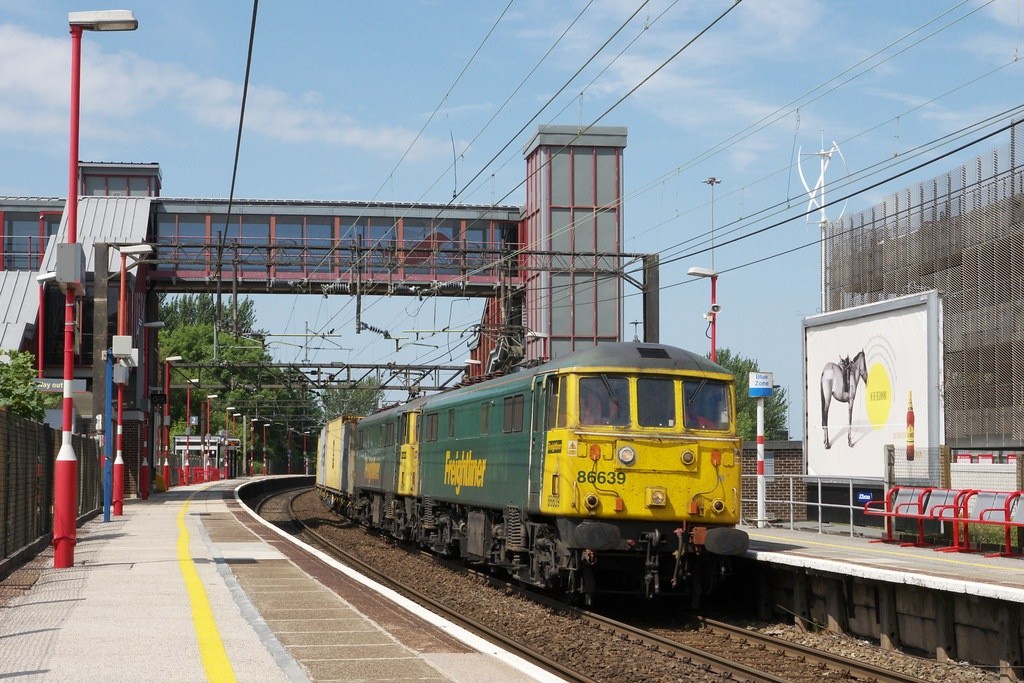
[232, 413, 241, 440]
[304, 431, 311, 471]
[206, 394, 218, 482]
[114, 244, 155, 517]
[250, 419, 259, 476]
[224, 406, 236, 480]
[49, 9, 139, 570]
[690, 176, 722, 363]
[142, 321, 165, 503]
[163, 355, 183, 492]
[37, 272, 58, 382]
[263, 424, 271, 476]
[288, 427, 295, 474]
[186, 378, 200, 485]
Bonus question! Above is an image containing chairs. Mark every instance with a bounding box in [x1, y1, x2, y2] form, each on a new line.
[864, 486, 935, 547]
[980, 491, 1024, 557]
[938, 489, 1018, 553]
[896, 488, 973, 551]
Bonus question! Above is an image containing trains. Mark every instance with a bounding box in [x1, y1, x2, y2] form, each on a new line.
[313, 340, 751, 622]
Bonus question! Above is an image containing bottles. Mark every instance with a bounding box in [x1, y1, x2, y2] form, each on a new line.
[906, 390, 915, 461]
